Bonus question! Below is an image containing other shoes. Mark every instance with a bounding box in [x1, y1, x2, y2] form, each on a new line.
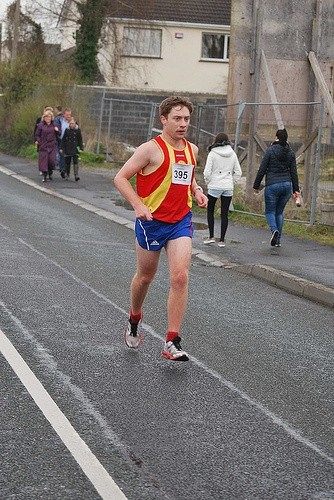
[40, 171, 43, 175]
[43, 178, 46, 182]
[49, 176, 52, 180]
[270, 229, 279, 246]
[55, 165, 61, 170]
[61, 171, 65, 178]
[275, 240, 281, 247]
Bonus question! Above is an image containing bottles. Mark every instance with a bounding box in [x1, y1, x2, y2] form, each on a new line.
[293, 190, 303, 208]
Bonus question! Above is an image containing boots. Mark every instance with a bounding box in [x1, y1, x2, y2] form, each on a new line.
[74, 164, 79, 181]
[67, 165, 70, 178]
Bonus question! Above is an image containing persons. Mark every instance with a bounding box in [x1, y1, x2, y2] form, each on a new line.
[112, 95, 211, 362]
[203, 131, 244, 248]
[31, 103, 85, 183]
[251, 127, 301, 248]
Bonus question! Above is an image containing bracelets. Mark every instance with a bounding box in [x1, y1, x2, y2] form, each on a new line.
[192, 186, 204, 197]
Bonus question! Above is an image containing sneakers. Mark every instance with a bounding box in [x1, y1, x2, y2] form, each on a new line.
[162, 336, 189, 361]
[204, 237, 215, 244]
[218, 241, 225, 248]
[125, 312, 143, 347]
